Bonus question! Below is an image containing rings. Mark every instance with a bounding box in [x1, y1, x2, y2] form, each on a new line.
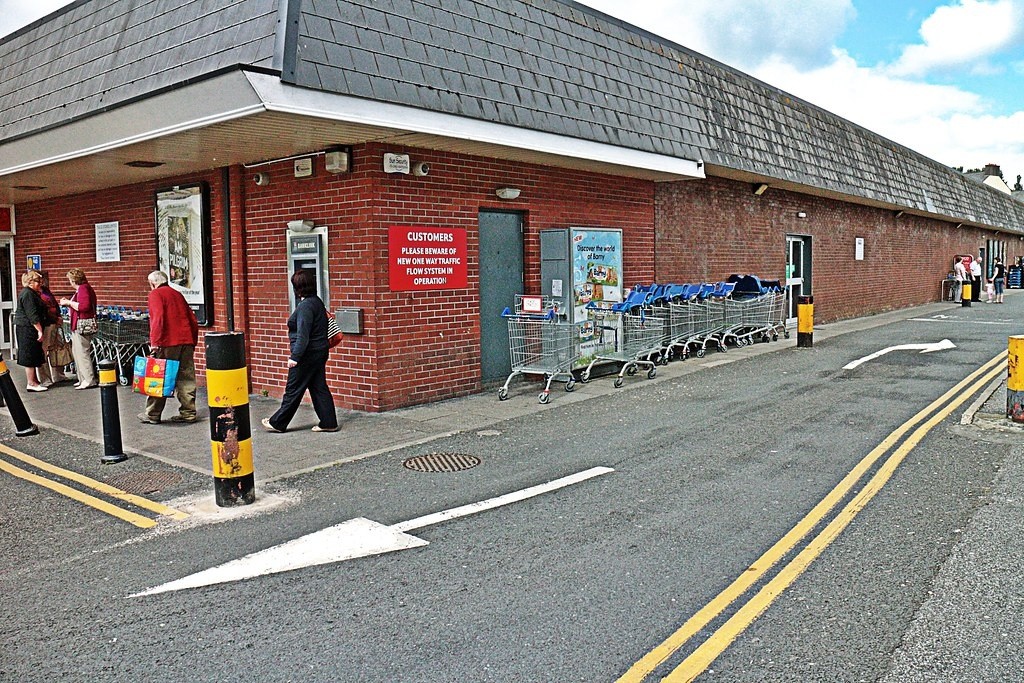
[293, 364, 295, 365]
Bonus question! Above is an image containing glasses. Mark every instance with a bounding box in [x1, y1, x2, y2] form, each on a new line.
[33, 280, 40, 283]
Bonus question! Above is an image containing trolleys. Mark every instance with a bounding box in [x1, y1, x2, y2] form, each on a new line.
[498, 294, 581, 404]
[58, 305, 153, 386]
[579, 273, 790, 388]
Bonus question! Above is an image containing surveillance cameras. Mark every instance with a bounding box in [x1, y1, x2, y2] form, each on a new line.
[414, 164, 429, 175]
[254, 172, 269, 186]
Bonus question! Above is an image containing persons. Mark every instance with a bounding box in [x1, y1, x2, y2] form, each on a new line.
[136, 271, 198, 424]
[985, 280, 995, 303]
[60, 268, 98, 389]
[954, 257, 966, 304]
[969, 257, 982, 302]
[1018, 261, 1023, 273]
[989, 257, 1005, 304]
[11, 269, 71, 391]
[262, 268, 338, 433]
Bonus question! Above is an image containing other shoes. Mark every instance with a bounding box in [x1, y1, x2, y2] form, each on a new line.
[262, 418, 281, 432]
[75, 381, 97, 390]
[974, 300, 981, 302]
[955, 302, 961, 303]
[26, 384, 48, 391]
[74, 381, 80, 386]
[171, 413, 196, 424]
[311, 425, 337, 432]
[137, 413, 161, 424]
[45, 378, 73, 388]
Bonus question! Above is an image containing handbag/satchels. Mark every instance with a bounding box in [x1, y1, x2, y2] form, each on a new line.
[76, 318, 99, 335]
[132, 350, 180, 398]
[48, 327, 74, 367]
[326, 310, 343, 348]
